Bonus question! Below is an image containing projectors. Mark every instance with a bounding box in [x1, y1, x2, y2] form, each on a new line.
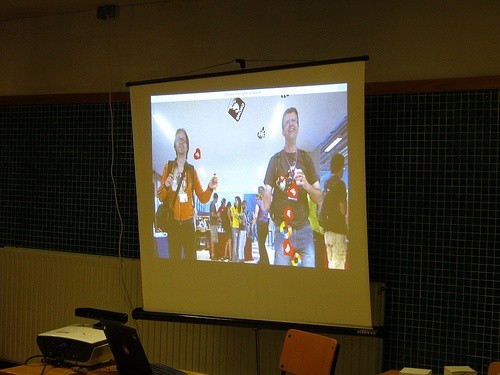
[36, 307, 128, 368]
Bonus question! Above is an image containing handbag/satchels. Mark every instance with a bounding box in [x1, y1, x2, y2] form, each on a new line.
[157, 205, 175, 232]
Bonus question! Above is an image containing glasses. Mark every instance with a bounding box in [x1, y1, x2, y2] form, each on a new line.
[176, 137, 186, 144]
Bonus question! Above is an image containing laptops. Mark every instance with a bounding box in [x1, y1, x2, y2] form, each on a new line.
[100, 320, 189, 375]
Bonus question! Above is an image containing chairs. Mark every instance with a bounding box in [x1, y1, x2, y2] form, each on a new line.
[278, 330, 340, 375]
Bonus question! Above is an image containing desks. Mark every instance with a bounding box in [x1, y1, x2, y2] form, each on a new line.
[0, 361, 209, 375]
